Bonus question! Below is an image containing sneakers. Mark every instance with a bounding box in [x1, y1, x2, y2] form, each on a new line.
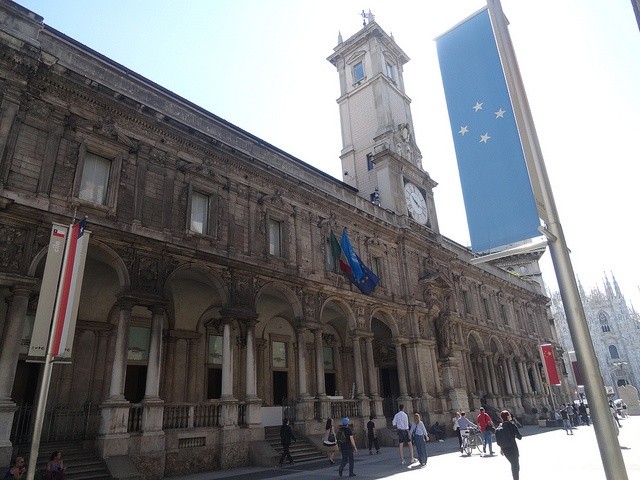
[401, 461, 407, 465]
[376, 451, 381, 455]
[329, 459, 335, 464]
[410, 459, 418, 463]
[369, 450, 373, 454]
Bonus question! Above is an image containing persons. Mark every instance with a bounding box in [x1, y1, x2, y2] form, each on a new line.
[367, 415, 382, 455]
[609, 400, 628, 427]
[477, 407, 496, 457]
[392, 404, 418, 465]
[322, 418, 339, 464]
[277, 418, 297, 467]
[452, 412, 464, 449]
[3, 457, 27, 480]
[408, 413, 429, 466]
[458, 412, 482, 456]
[429, 421, 447, 443]
[43, 449, 66, 480]
[494, 410, 522, 480]
[335, 418, 359, 477]
[555, 403, 589, 435]
[500, 408, 523, 430]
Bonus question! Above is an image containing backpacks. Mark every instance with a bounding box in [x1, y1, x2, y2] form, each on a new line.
[495, 424, 515, 450]
[336, 428, 350, 451]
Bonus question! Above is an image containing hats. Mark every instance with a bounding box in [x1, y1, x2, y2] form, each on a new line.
[342, 417, 350, 425]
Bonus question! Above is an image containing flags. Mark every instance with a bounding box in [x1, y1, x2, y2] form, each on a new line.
[330, 230, 351, 274]
[341, 228, 363, 282]
[351, 267, 386, 294]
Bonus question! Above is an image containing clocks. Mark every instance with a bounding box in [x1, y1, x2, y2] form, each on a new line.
[405, 182, 428, 225]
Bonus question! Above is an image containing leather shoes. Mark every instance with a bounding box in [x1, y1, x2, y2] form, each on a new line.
[349, 474, 356, 477]
[338, 468, 343, 477]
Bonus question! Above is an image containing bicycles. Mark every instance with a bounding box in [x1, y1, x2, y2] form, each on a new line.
[463, 427, 483, 454]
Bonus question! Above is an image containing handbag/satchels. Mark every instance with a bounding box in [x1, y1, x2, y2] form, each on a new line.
[411, 424, 417, 444]
[485, 413, 496, 437]
[328, 425, 335, 442]
[452, 424, 457, 430]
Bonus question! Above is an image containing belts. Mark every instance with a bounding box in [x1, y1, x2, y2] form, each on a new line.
[461, 429, 467, 430]
